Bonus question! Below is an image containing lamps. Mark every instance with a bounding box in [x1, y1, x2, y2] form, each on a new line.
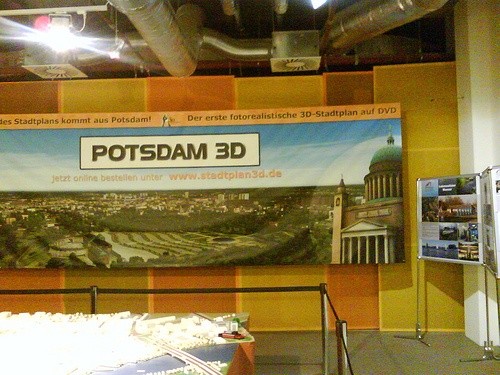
[47, 12, 77, 55]
